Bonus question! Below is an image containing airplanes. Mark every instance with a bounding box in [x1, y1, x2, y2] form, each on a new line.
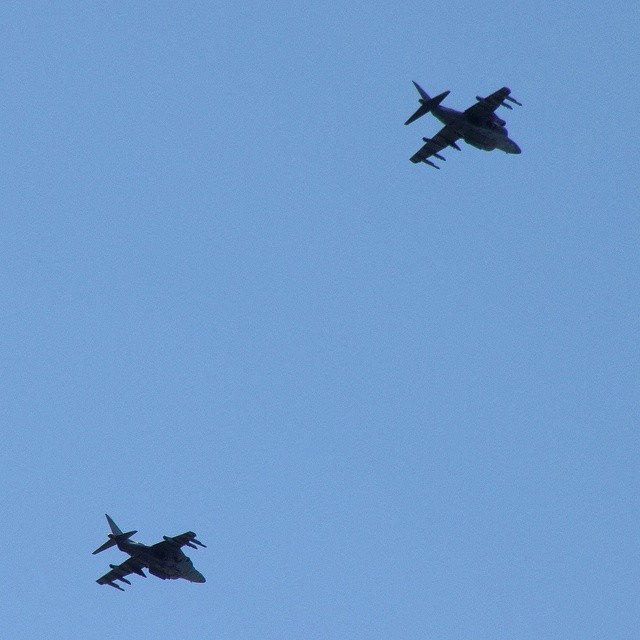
[91, 513, 207, 591]
[403, 80, 522, 169]
